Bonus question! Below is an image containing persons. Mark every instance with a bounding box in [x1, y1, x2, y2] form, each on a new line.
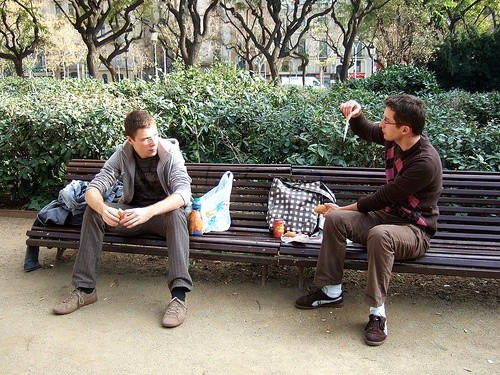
[294, 95, 442, 346]
[53, 110, 193, 327]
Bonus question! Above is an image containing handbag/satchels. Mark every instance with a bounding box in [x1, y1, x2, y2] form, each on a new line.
[266, 176, 337, 235]
[195, 171, 233, 234]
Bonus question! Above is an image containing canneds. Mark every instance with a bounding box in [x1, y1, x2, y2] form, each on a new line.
[274, 219, 284, 238]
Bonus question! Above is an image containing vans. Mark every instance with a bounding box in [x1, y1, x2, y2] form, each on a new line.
[281, 77, 326, 91]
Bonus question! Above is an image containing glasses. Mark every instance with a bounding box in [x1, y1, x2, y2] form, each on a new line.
[382, 115, 410, 129]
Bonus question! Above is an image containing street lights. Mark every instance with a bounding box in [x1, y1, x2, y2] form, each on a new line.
[149, 24, 159, 79]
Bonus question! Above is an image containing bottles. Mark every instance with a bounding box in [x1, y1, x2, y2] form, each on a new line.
[187, 203, 203, 236]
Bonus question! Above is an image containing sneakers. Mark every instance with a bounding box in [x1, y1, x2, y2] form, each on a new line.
[295, 288, 344, 309]
[53, 287, 98, 315]
[364, 312, 387, 346]
[163, 296, 187, 327]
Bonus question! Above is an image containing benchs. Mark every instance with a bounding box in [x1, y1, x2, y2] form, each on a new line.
[279, 164, 500, 290]
[25, 158, 292, 284]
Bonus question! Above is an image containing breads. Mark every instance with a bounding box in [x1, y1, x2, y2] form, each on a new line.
[284, 231, 296, 237]
[314, 205, 329, 214]
[117, 208, 126, 219]
